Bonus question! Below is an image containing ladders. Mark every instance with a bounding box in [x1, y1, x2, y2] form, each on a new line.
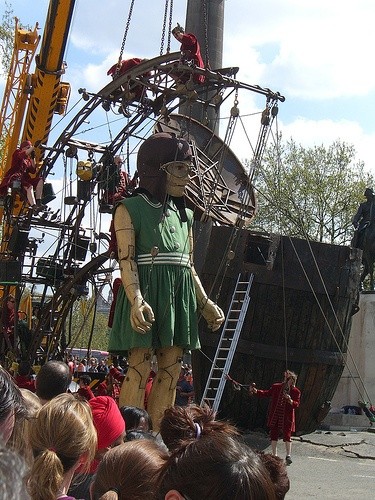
[200, 273, 254, 422]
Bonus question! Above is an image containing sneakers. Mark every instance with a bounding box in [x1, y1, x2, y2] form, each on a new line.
[286, 455, 292, 464]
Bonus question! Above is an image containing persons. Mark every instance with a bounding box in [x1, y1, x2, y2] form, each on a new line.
[106, 57, 153, 118]
[351, 188, 374, 289]
[22, 355, 230, 454]
[104, 156, 135, 204]
[90, 440, 167, 499]
[0, 295, 21, 356]
[0, 141, 42, 214]
[26, 393, 97, 500]
[250, 371, 300, 464]
[167, 27, 205, 85]
[0, 365, 28, 499]
[106, 132, 225, 409]
[156, 404, 291, 500]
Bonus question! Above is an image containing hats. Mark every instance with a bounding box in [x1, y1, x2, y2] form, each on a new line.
[88, 396, 125, 449]
[114, 155, 125, 165]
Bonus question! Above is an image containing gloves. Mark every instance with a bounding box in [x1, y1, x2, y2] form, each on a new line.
[249, 387, 257, 395]
[285, 394, 292, 403]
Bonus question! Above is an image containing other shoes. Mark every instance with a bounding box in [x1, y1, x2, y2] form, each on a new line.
[102, 101, 111, 111]
[37, 200, 45, 211]
[32, 205, 40, 214]
[118, 105, 131, 118]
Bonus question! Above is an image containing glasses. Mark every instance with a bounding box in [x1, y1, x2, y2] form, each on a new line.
[25, 146, 30, 149]
